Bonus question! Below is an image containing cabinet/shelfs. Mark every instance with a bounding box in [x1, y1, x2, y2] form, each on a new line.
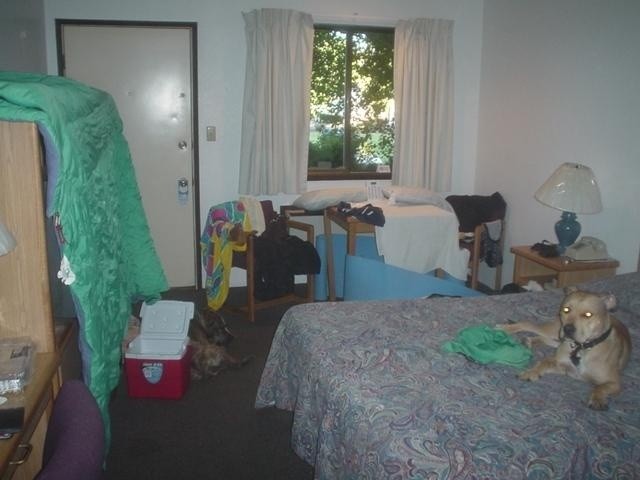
[0, 121, 78, 479]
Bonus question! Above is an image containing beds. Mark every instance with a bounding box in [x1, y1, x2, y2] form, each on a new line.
[254, 272, 640, 480]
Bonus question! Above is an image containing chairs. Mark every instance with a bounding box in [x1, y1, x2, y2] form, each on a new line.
[435, 220, 505, 293]
[209, 200, 314, 322]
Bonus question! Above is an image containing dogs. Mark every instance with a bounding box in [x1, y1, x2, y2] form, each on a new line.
[494, 286, 631, 411]
[190, 308, 258, 382]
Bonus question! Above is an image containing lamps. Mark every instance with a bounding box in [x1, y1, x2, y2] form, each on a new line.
[533, 163, 603, 247]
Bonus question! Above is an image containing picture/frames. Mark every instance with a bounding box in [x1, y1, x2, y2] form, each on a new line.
[280, 205, 375, 292]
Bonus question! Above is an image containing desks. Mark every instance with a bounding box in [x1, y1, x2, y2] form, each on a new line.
[324, 203, 377, 303]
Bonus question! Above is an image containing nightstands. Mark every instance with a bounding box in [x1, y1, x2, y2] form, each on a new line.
[511, 244, 619, 291]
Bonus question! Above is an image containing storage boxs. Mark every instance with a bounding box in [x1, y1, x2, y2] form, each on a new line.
[122, 297, 195, 400]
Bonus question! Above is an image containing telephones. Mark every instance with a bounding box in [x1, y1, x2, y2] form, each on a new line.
[568, 236, 608, 261]
